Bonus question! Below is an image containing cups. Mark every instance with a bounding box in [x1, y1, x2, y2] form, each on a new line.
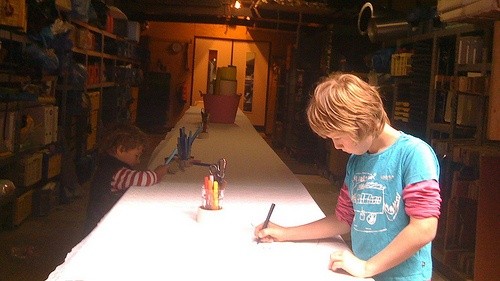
[200, 185, 225, 210]
[177, 144, 192, 160]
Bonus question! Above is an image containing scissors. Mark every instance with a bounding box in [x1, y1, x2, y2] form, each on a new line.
[208, 157, 226, 185]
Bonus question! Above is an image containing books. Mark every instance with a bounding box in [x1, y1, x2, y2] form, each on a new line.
[392, 35, 491, 276]
[1, 105, 63, 227]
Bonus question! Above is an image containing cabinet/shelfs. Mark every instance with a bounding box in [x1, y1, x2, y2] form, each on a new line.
[346, 21, 492, 281]
[0, 0, 144, 214]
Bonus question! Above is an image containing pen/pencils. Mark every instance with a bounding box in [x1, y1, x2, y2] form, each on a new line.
[256, 203, 275, 245]
[165, 144, 177, 165]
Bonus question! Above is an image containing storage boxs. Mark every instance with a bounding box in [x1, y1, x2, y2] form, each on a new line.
[0, 92, 100, 229]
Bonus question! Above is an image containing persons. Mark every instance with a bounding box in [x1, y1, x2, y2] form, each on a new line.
[252, 72, 442, 281]
[85, 127, 171, 236]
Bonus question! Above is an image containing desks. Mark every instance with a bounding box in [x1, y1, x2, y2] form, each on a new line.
[46, 101, 376, 281]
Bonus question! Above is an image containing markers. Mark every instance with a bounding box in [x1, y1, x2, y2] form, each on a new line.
[175, 126, 198, 160]
[199, 175, 226, 210]
